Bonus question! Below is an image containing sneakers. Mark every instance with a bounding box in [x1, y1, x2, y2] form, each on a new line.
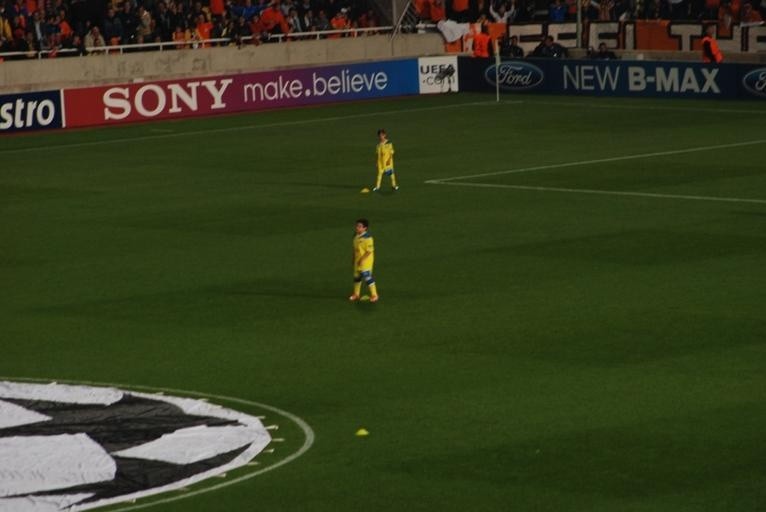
[349, 294, 378, 303]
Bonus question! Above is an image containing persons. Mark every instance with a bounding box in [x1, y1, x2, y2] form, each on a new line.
[373, 128, 399, 192]
[1, 1, 766, 61]
[348, 221, 380, 303]
